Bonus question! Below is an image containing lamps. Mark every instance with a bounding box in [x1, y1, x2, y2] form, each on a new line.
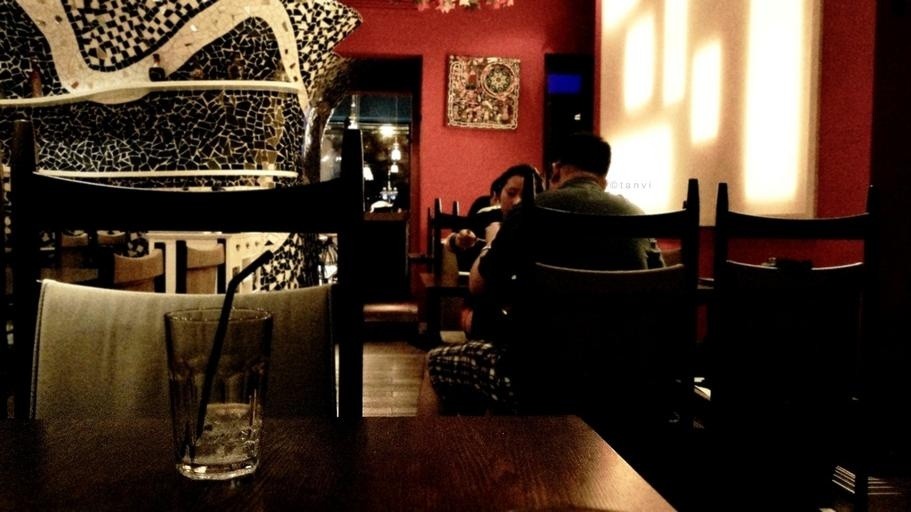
[363, 91, 402, 181]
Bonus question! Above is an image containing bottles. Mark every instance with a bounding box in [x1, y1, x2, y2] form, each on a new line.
[380, 187, 398, 202]
[257, 161, 272, 186]
[28, 57, 43, 98]
[149, 54, 167, 81]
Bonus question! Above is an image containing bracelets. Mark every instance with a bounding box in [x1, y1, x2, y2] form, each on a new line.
[480, 246, 492, 251]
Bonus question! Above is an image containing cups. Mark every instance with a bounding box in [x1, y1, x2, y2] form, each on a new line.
[164, 307, 274, 484]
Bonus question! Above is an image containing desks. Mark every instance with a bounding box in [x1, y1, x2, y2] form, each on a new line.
[0, 415, 679, 512]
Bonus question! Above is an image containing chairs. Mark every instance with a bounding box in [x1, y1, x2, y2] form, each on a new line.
[98, 242, 166, 292]
[15, 282, 362, 420]
[176, 238, 226, 293]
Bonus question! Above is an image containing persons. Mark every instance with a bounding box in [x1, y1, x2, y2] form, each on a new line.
[442, 163, 542, 333]
[321, 131, 389, 206]
[417, 132, 666, 419]
[490, 178, 502, 206]
